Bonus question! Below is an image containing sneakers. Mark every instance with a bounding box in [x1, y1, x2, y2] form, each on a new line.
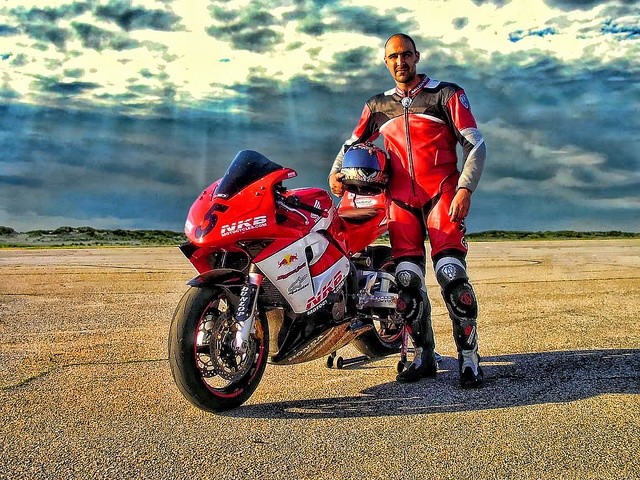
[458, 350, 484, 389]
[396, 347, 438, 383]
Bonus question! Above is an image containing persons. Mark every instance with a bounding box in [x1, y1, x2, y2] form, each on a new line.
[326, 33, 487, 389]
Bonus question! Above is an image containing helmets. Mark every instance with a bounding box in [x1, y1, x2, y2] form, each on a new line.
[340, 141, 389, 197]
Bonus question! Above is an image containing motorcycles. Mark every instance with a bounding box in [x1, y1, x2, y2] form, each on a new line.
[168, 150, 407, 412]
